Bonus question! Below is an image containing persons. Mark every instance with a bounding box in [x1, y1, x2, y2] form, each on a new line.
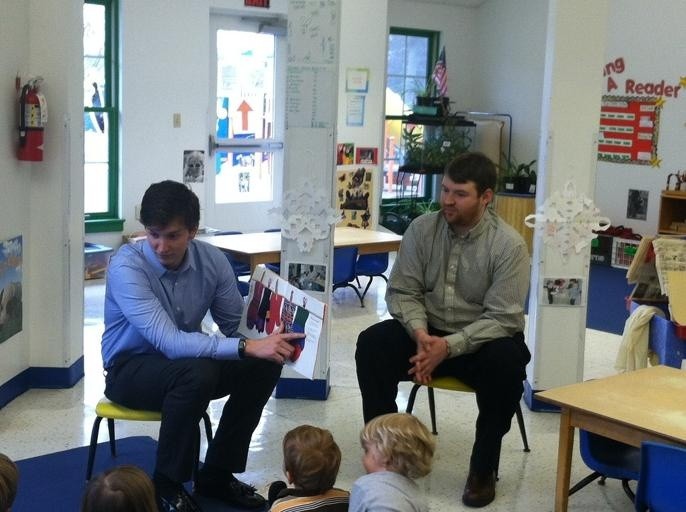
[0, 453, 20, 512]
[101, 180, 306, 512]
[348, 413, 436, 512]
[80, 465, 159, 512]
[547, 279, 580, 305]
[184, 151, 204, 182]
[337, 144, 353, 164]
[267, 425, 350, 512]
[355, 153, 532, 507]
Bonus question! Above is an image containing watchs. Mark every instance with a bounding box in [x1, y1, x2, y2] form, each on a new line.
[238, 337, 247, 360]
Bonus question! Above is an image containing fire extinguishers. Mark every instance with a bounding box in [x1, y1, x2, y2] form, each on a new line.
[16, 71, 48, 162]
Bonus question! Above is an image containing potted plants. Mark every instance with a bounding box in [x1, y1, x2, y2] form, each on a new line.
[495, 152, 528, 193]
[401, 78, 450, 115]
[519, 162, 536, 193]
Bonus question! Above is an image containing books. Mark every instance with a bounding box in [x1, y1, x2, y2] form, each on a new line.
[237, 263, 333, 382]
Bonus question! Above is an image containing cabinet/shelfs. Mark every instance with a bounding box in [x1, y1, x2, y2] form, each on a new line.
[657, 193, 686, 237]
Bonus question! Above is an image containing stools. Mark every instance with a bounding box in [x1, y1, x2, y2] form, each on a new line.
[84, 393, 214, 486]
[406, 374, 532, 454]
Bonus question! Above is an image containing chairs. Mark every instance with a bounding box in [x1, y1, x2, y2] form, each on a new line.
[353, 248, 390, 301]
[332, 246, 365, 308]
[213, 229, 256, 297]
[262, 228, 281, 273]
[567, 429, 643, 505]
[634, 440, 686, 511]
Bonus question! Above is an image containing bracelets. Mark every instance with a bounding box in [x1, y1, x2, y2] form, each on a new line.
[446, 341, 451, 358]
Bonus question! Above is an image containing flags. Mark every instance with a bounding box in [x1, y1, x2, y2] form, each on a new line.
[431, 47, 448, 97]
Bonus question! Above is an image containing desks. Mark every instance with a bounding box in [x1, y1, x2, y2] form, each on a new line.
[535, 363, 685, 512]
[193, 224, 403, 281]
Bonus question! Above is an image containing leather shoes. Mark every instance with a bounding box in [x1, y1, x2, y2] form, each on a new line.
[463, 459, 498, 507]
[151, 476, 202, 511]
[197, 464, 266, 510]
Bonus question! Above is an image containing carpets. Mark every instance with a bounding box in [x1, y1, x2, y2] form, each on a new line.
[526, 264, 634, 335]
[3, 434, 204, 511]
